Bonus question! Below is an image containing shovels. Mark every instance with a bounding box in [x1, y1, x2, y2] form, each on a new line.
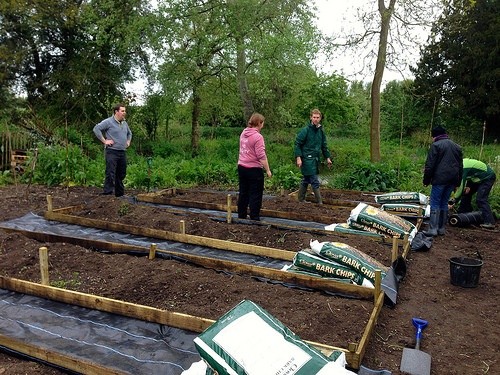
[400, 318, 432, 375]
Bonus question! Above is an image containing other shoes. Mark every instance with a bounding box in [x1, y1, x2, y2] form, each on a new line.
[480, 223, 495, 229]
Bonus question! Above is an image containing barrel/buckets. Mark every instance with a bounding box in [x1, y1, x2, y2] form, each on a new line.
[448, 248, 483, 288]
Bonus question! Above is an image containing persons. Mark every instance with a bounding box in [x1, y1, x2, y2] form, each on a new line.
[237, 113, 272, 221]
[92, 105, 133, 198]
[447, 158, 495, 229]
[293, 109, 332, 208]
[422, 125, 463, 236]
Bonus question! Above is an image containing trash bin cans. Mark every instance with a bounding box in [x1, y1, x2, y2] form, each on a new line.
[448, 256, 483, 288]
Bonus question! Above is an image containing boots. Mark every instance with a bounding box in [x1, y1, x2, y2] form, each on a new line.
[297, 186, 311, 205]
[437, 208, 448, 236]
[312, 188, 323, 207]
[422, 208, 440, 237]
